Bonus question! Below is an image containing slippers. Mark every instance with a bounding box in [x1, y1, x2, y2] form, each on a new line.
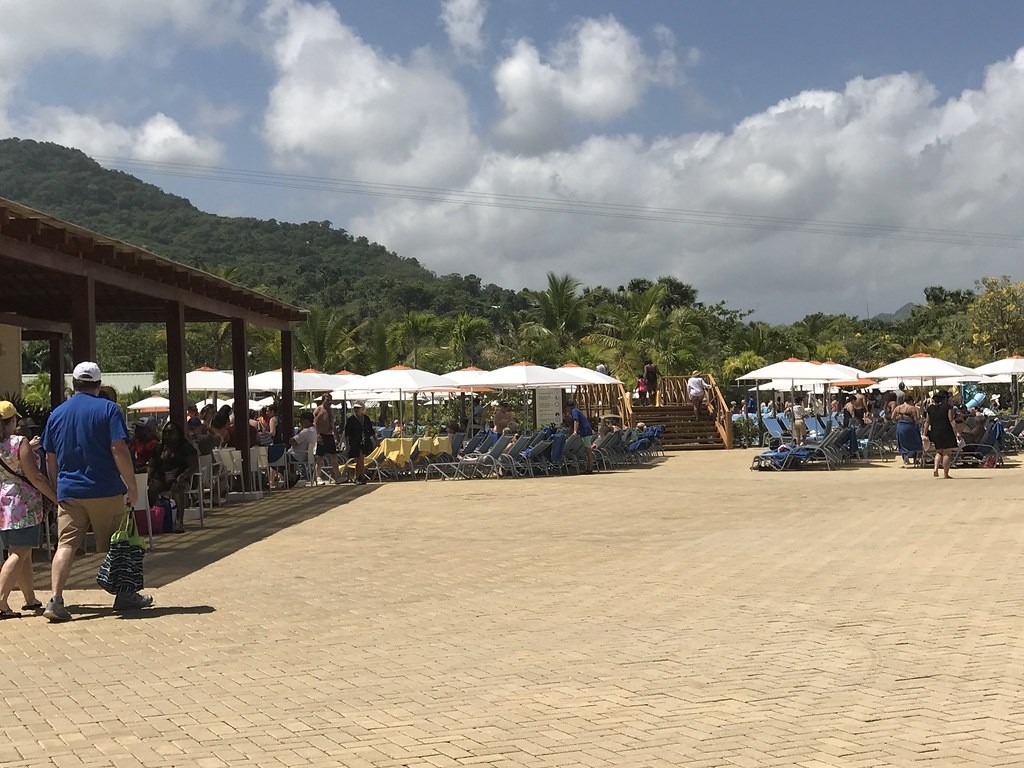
[21, 600, 43, 610]
[173, 525, 185, 532]
[0, 609, 21, 620]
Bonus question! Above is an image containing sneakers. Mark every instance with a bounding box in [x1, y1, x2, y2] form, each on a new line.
[43, 595, 72, 621]
[113, 592, 153, 610]
[355, 477, 366, 485]
[335, 476, 348, 484]
[317, 476, 325, 485]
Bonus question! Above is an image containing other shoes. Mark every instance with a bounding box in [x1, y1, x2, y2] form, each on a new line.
[289, 475, 300, 488]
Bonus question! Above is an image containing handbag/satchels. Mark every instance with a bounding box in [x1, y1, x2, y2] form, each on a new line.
[136, 498, 172, 533]
[257, 421, 273, 446]
[43, 494, 53, 513]
[96, 505, 144, 595]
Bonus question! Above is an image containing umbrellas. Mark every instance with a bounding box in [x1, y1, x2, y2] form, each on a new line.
[127, 361, 624, 438]
[734, 352, 1024, 443]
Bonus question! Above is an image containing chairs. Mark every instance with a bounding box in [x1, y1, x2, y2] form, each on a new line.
[42, 421, 668, 562]
[751, 401, 1024, 472]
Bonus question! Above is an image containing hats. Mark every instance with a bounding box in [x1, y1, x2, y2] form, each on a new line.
[693, 371, 702, 377]
[297, 412, 315, 421]
[354, 400, 366, 407]
[562, 400, 576, 407]
[500, 400, 510, 407]
[0, 401, 22, 419]
[132, 417, 151, 427]
[73, 362, 101, 382]
[935, 390, 951, 397]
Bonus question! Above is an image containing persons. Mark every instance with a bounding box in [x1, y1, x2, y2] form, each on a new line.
[633, 358, 666, 407]
[730, 380, 1001, 478]
[37, 362, 154, 623]
[120, 394, 646, 533]
[687, 370, 717, 422]
[0, 400, 56, 620]
[922, 390, 961, 478]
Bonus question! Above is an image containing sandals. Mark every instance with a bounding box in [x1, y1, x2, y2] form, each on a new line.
[581, 470, 594, 475]
